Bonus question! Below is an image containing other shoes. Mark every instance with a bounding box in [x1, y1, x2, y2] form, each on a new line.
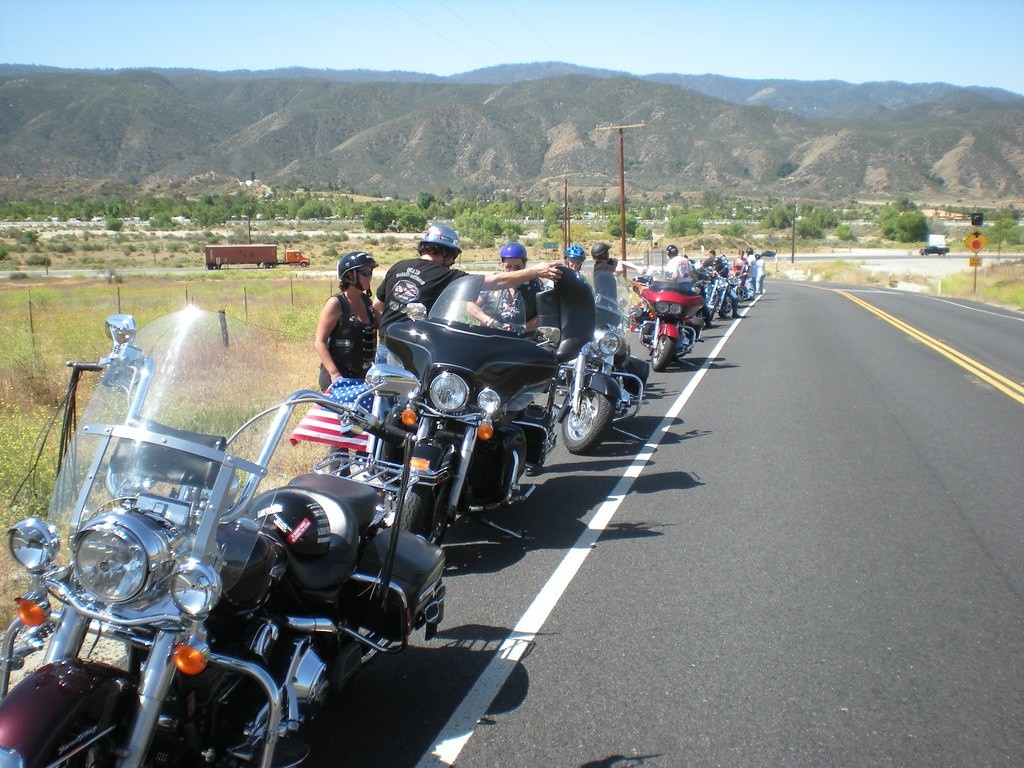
[704, 318, 712, 327]
[732, 314, 741, 318]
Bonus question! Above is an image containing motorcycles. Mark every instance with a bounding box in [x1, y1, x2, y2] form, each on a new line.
[1, 251, 794, 768]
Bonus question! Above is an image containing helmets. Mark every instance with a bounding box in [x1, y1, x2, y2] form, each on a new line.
[739, 251, 744, 255]
[714, 258, 721, 264]
[756, 254, 760, 259]
[666, 245, 678, 258]
[421, 225, 462, 254]
[565, 245, 586, 262]
[591, 242, 610, 259]
[251, 493, 331, 555]
[746, 248, 752, 255]
[337, 251, 380, 280]
[501, 243, 527, 259]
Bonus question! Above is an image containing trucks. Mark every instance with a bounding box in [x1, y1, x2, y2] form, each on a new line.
[206, 244, 310, 270]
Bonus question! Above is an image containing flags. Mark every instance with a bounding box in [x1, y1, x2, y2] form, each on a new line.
[290, 377, 375, 454]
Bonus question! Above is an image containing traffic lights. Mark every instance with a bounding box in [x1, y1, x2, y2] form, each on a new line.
[972, 213, 983, 225]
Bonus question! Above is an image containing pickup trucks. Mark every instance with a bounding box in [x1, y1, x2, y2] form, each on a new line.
[919, 246, 949, 256]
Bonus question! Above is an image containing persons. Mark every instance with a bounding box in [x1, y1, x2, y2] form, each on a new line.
[547, 245, 588, 288]
[665, 244, 712, 329]
[591, 242, 644, 311]
[466, 242, 552, 476]
[704, 250, 742, 319]
[315, 251, 379, 477]
[373, 225, 563, 420]
[736, 247, 766, 302]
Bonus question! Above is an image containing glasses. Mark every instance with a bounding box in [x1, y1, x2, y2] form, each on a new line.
[449, 253, 459, 261]
[356, 270, 373, 277]
